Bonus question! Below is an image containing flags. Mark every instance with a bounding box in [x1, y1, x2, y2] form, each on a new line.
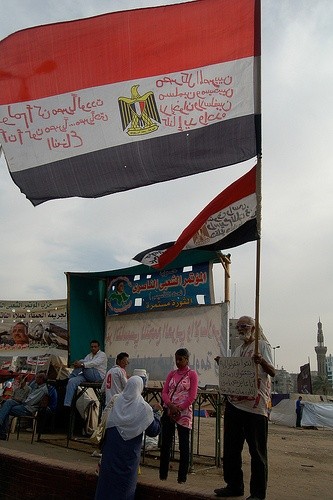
[133, 164, 261, 270]
[0, 0, 261, 208]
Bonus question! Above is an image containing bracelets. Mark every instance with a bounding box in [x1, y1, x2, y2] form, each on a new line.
[81, 362, 84, 367]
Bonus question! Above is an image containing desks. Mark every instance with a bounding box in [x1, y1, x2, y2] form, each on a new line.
[38, 381, 221, 474]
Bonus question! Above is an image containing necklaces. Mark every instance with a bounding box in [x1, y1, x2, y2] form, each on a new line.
[239, 343, 252, 357]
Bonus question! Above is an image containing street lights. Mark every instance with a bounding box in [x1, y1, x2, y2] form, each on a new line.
[273, 345, 280, 394]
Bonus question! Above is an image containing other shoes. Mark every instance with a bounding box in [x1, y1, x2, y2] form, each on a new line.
[214, 487, 244, 496]
[246, 495, 264, 500]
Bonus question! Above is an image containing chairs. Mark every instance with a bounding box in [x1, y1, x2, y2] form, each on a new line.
[7, 394, 51, 444]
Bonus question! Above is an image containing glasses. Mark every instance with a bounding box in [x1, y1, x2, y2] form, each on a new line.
[121, 360, 130, 364]
[235, 324, 252, 329]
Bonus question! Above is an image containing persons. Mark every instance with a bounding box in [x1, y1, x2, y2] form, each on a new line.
[214, 316, 273, 500]
[96, 375, 165, 500]
[0, 372, 58, 440]
[100, 352, 129, 409]
[159, 349, 197, 483]
[295, 395, 306, 429]
[63, 340, 107, 407]
